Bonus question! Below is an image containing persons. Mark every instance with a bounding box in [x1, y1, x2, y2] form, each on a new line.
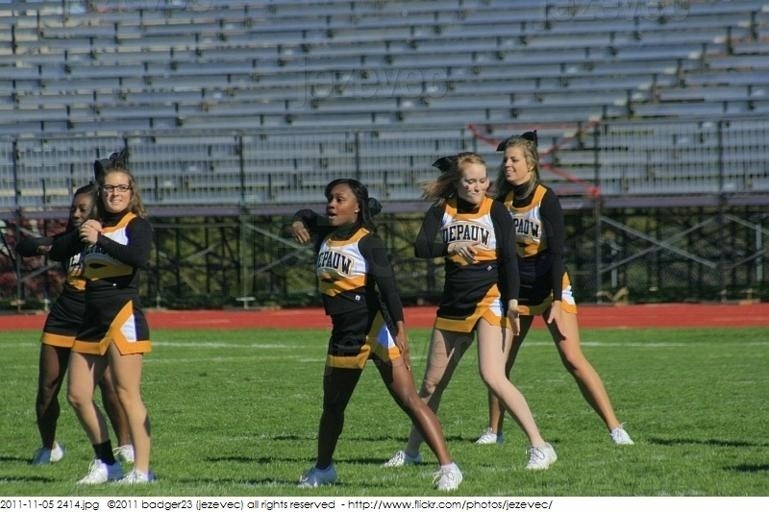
[15, 184, 134, 463]
[57, 149, 152, 487]
[475, 131, 636, 447]
[292, 180, 463, 492]
[379, 153, 557, 473]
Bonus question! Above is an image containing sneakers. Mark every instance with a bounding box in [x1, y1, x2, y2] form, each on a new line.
[77, 459, 124, 485]
[432, 462, 463, 490]
[33, 441, 64, 465]
[610, 428, 634, 444]
[476, 427, 504, 446]
[384, 451, 423, 467]
[298, 462, 337, 488]
[525, 442, 558, 469]
[115, 469, 154, 485]
[114, 445, 136, 462]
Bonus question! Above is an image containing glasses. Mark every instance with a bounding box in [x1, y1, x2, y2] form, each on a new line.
[100, 184, 131, 191]
